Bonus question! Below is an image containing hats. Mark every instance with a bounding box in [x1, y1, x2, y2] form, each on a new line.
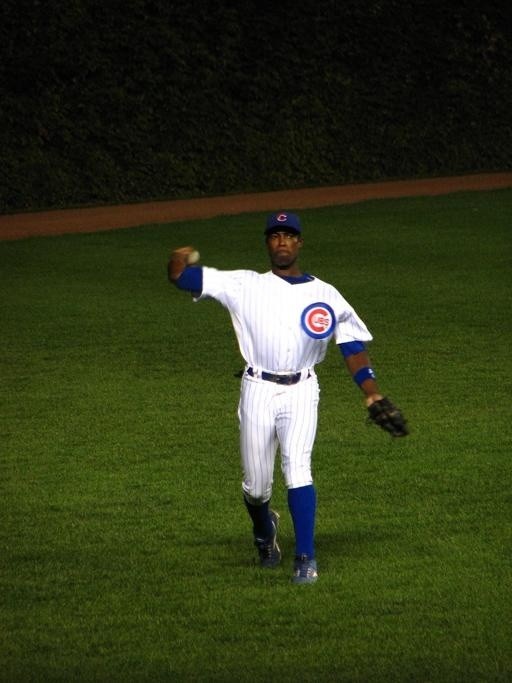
[264, 212, 300, 235]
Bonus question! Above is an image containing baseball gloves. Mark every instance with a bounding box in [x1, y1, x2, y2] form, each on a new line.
[366, 394, 410, 437]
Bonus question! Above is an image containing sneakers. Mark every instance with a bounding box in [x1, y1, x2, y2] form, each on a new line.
[293, 554, 318, 584]
[254, 509, 281, 568]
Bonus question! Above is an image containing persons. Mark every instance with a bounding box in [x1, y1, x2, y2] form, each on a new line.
[167, 210, 408, 584]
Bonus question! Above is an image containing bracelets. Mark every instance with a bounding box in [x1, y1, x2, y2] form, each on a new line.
[352, 366, 378, 385]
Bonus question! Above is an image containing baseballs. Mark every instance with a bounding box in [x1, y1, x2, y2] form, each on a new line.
[187, 250, 201, 264]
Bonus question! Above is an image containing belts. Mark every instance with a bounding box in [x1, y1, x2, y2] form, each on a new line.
[247, 367, 311, 384]
